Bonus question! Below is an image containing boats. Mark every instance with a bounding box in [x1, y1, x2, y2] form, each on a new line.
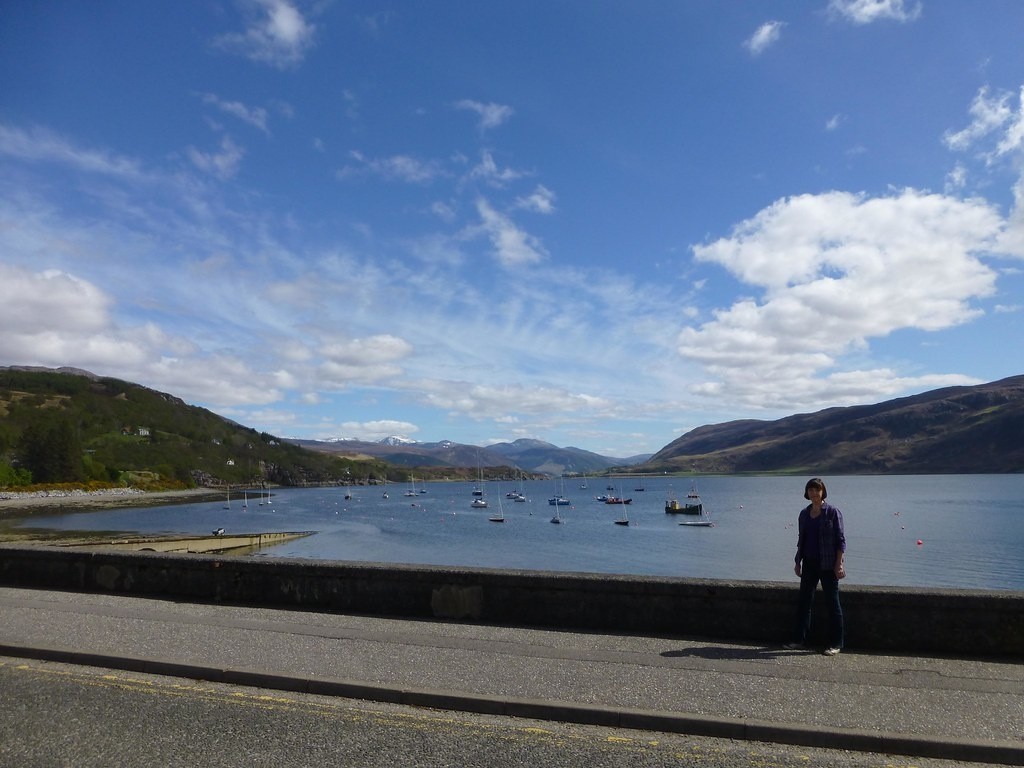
[597, 495, 614, 501]
[606, 497, 633, 504]
[634, 488, 646, 491]
[344, 487, 352, 500]
[665, 500, 703, 515]
[679, 521, 711, 526]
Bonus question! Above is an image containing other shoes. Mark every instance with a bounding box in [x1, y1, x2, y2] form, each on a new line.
[783, 644, 804, 650]
[824, 646, 840, 655]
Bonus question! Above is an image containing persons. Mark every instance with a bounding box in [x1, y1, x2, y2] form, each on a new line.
[782, 478, 846, 656]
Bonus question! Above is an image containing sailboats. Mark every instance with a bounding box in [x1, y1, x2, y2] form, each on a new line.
[470, 467, 490, 509]
[579, 471, 588, 490]
[259, 486, 265, 506]
[242, 490, 249, 507]
[688, 469, 701, 498]
[420, 476, 427, 493]
[488, 482, 505, 522]
[550, 493, 561, 524]
[222, 486, 231, 509]
[471, 451, 483, 495]
[514, 472, 526, 502]
[410, 474, 419, 496]
[613, 482, 630, 525]
[382, 475, 390, 499]
[267, 483, 272, 504]
[607, 469, 614, 490]
[548, 470, 571, 505]
[403, 481, 411, 496]
[506, 468, 519, 499]
